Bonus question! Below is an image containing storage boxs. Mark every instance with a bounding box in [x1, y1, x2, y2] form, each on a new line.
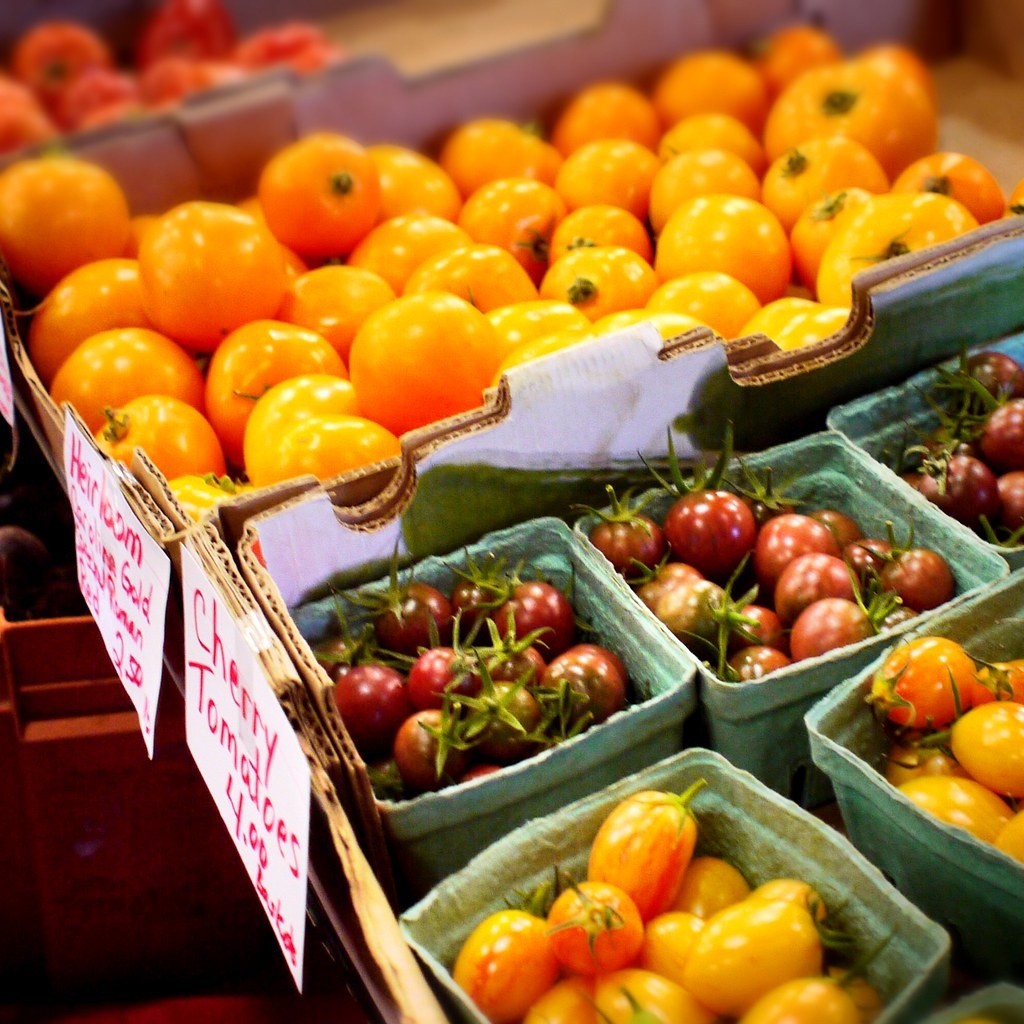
[2, 2, 1023, 1024]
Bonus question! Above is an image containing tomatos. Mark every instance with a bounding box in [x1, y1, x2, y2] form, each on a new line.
[0, 1, 1022, 1024]
